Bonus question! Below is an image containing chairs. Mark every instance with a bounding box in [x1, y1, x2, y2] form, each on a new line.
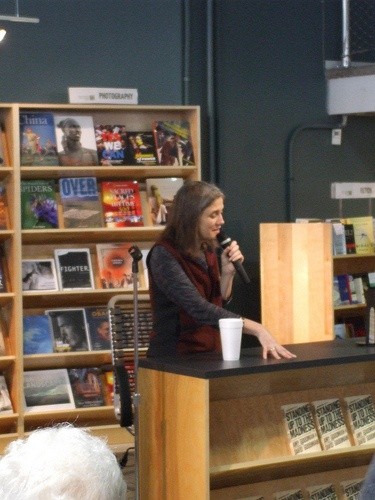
[108, 294, 152, 470]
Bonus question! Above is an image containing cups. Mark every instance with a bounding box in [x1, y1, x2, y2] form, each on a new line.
[219, 318, 243, 361]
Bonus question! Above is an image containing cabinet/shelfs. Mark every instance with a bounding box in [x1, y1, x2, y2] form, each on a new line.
[0, 103, 375, 500]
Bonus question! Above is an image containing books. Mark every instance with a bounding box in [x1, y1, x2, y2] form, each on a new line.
[344, 394, 375, 446]
[236, 477, 364, 500]
[24, 305, 119, 410]
[20, 113, 197, 229]
[97, 242, 140, 290]
[138, 242, 157, 289]
[312, 398, 352, 451]
[296, 219, 375, 340]
[280, 402, 322, 456]
[22, 247, 96, 292]
[0, 116, 14, 416]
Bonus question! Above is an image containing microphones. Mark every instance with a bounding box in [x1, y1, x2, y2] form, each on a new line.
[216, 232, 251, 284]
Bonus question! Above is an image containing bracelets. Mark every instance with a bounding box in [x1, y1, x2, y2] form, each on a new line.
[239, 317, 246, 334]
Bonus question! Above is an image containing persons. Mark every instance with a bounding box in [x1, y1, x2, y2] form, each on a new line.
[45, 138, 56, 160]
[94, 319, 110, 350]
[95, 125, 122, 166]
[0, 422, 126, 500]
[21, 127, 42, 161]
[147, 180, 297, 359]
[129, 134, 156, 166]
[57, 118, 98, 167]
[100, 270, 139, 289]
[147, 184, 168, 226]
[57, 310, 88, 351]
